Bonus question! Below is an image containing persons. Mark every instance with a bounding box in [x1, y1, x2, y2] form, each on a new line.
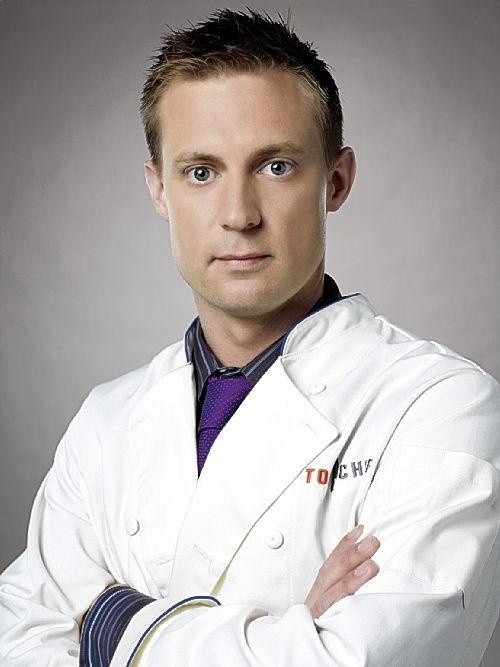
[0, 4, 498, 667]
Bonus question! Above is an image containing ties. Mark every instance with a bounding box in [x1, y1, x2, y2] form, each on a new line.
[195, 376, 254, 478]
